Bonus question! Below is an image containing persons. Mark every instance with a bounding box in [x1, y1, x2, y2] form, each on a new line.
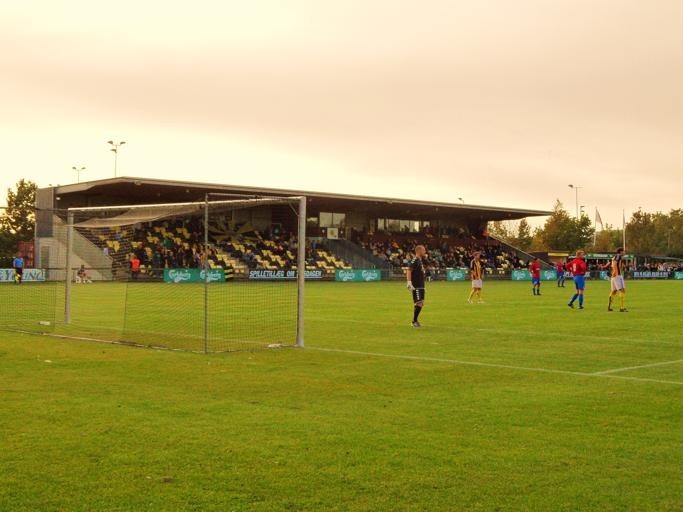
[13, 251, 24, 283]
[468, 252, 483, 304]
[566, 250, 587, 309]
[557, 257, 566, 287]
[101, 218, 528, 280]
[550, 261, 682, 279]
[78, 263, 92, 283]
[608, 247, 627, 312]
[529, 257, 542, 294]
[407, 245, 426, 327]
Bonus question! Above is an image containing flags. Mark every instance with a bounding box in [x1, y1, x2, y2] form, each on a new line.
[595, 211, 603, 224]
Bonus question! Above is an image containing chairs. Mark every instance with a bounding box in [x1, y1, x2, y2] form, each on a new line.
[76, 226, 532, 280]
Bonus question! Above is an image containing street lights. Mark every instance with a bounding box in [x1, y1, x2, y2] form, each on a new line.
[107, 139, 126, 175]
[72, 165, 87, 184]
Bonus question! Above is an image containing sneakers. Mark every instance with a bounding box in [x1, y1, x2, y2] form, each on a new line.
[478, 301, 484, 304]
[568, 303, 573, 308]
[578, 306, 583, 309]
[467, 299, 473, 303]
[608, 309, 612, 311]
[411, 320, 420, 328]
[620, 308, 625, 311]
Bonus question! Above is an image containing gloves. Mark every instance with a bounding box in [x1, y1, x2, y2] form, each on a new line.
[406, 281, 415, 291]
